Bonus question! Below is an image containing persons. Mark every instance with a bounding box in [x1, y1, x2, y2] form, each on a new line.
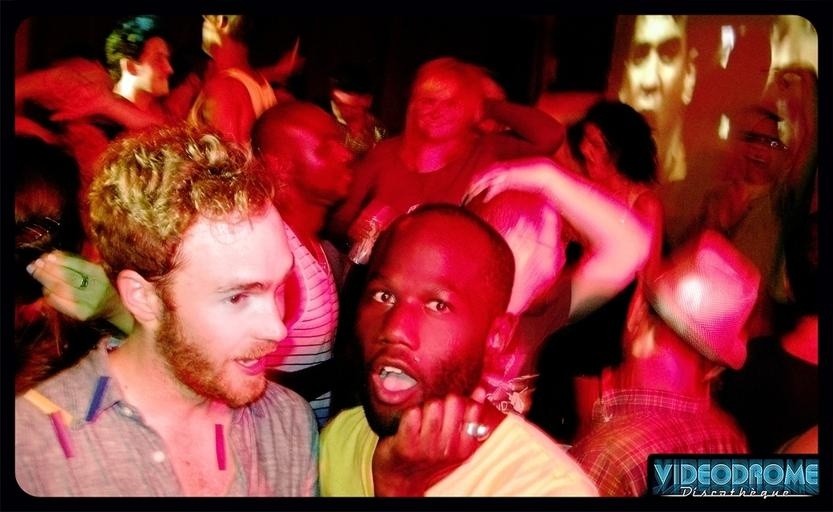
[14, 13, 817, 495]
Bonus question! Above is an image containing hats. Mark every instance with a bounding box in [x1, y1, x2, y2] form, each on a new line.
[644, 231, 759, 371]
